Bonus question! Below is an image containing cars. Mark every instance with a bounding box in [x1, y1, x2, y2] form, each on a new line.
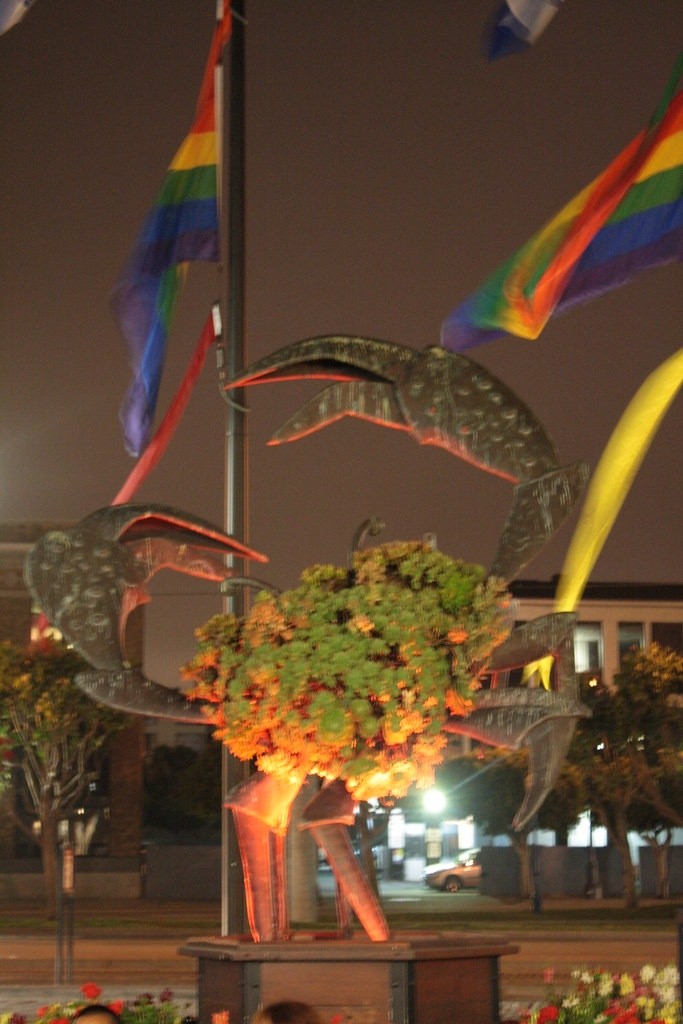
[421, 846, 482, 895]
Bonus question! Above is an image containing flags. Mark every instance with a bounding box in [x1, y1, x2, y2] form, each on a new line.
[107, 1, 233, 457]
[479, 0, 565, 60]
[442, 52, 683, 353]
